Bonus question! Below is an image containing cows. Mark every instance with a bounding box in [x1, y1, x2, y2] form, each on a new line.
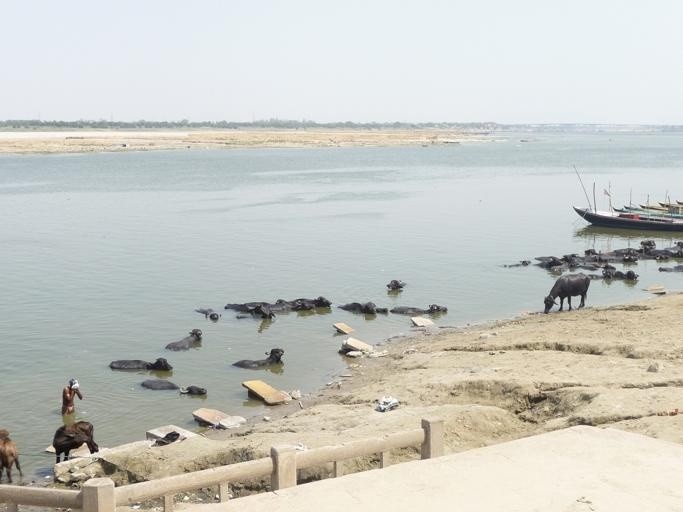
[0, 429, 24, 483]
[532, 238, 683, 280]
[338, 300, 449, 316]
[385, 279, 407, 290]
[140, 378, 208, 395]
[499, 259, 531, 269]
[107, 357, 174, 373]
[222, 296, 331, 320]
[52, 420, 101, 464]
[164, 329, 204, 352]
[232, 348, 285, 369]
[193, 306, 222, 320]
[542, 269, 590, 314]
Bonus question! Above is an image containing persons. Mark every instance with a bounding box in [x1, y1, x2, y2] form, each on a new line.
[62, 378, 83, 414]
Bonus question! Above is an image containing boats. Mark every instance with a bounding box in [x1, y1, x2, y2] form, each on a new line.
[571, 162, 683, 229]
